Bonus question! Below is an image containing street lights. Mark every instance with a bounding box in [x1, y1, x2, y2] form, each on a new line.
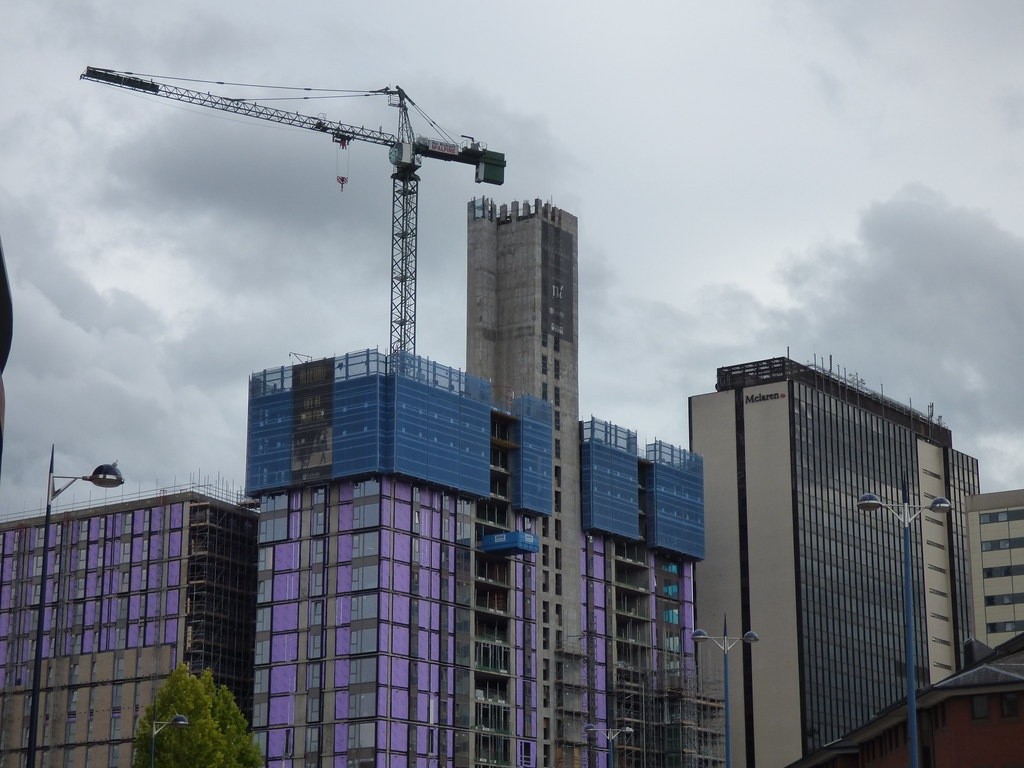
[586, 722, 634, 768]
[150, 705, 189, 768]
[857, 492, 956, 767]
[690, 611, 761, 767]
[25, 443, 126, 765]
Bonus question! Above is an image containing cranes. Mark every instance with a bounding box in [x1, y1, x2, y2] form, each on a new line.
[79, 63, 506, 379]
